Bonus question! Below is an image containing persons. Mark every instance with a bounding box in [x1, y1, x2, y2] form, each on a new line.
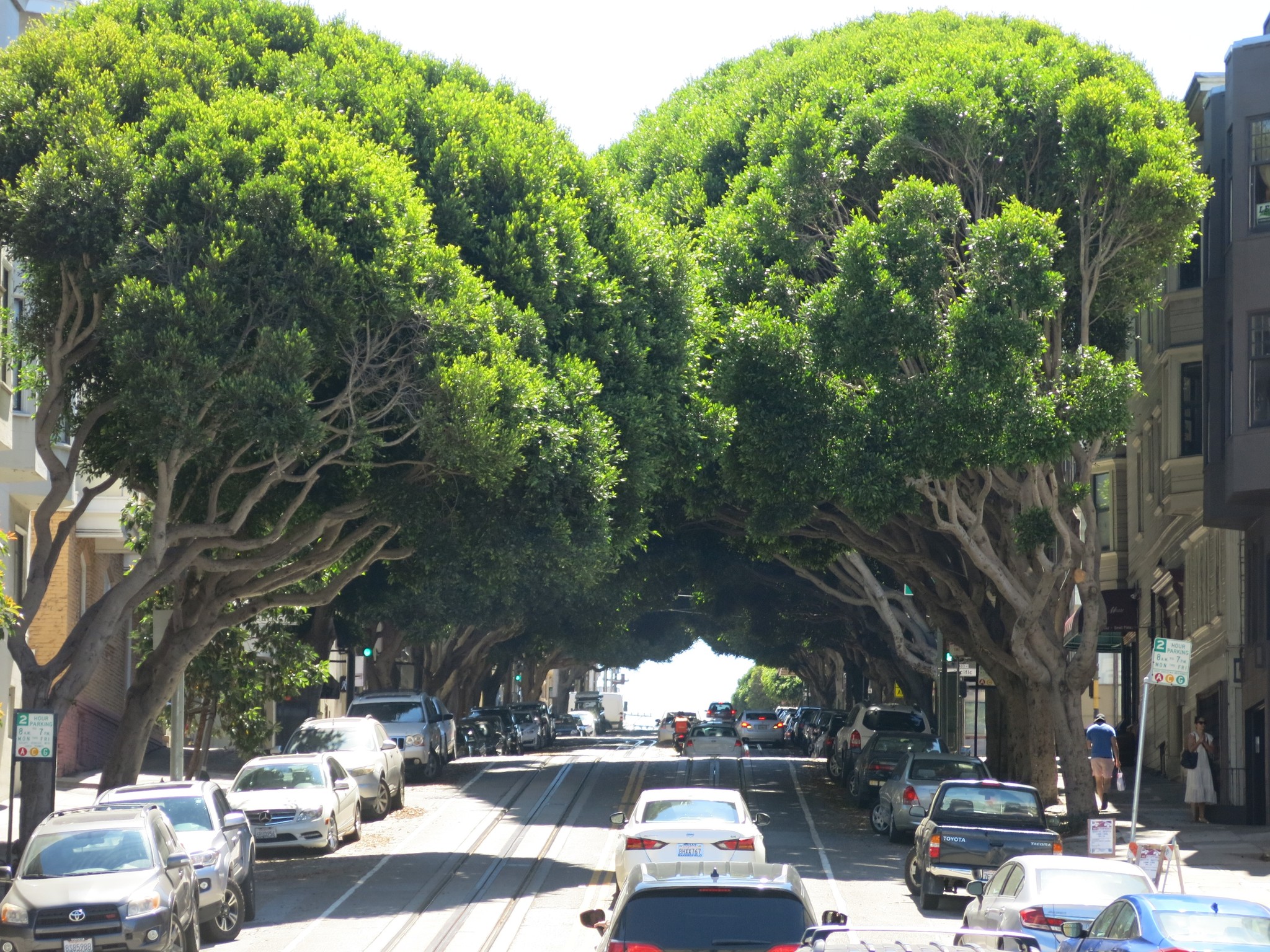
[1181, 714, 1217, 825]
[1085, 713, 1121, 810]
[671, 711, 691, 747]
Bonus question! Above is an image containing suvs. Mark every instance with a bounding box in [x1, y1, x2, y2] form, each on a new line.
[825, 700, 933, 785]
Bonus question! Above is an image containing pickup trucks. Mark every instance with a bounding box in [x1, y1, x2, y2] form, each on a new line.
[904, 779, 1064, 911]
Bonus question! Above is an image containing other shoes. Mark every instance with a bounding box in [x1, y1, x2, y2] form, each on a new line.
[1101, 793, 1108, 810]
[1198, 817, 1211, 824]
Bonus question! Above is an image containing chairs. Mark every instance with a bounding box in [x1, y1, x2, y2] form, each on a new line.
[949, 799, 974, 811]
[916, 769, 935, 778]
[960, 773, 979, 779]
[1005, 803, 1028, 814]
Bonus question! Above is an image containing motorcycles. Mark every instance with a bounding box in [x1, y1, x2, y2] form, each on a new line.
[672, 732, 688, 755]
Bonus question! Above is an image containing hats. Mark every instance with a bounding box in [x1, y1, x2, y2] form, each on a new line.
[1096, 713, 1105, 721]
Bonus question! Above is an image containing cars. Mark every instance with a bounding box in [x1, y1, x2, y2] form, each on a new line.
[429, 690, 624, 765]
[844, 730, 957, 801]
[773, 705, 851, 759]
[733, 710, 785, 749]
[0, 801, 202, 952]
[869, 748, 995, 844]
[346, 688, 454, 782]
[677, 723, 750, 756]
[281, 714, 406, 820]
[224, 751, 362, 854]
[1055, 893, 1270, 952]
[609, 787, 771, 892]
[703, 701, 738, 723]
[579, 860, 850, 952]
[961, 854, 1158, 952]
[88, 769, 258, 943]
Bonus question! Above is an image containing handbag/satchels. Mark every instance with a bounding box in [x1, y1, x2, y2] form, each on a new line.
[1180, 750, 1199, 769]
[1116, 768, 1126, 791]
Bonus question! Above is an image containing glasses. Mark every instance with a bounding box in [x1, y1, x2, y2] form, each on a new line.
[1197, 721, 1206, 725]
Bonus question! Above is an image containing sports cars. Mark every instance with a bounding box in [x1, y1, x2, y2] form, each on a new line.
[654, 711, 704, 748]
